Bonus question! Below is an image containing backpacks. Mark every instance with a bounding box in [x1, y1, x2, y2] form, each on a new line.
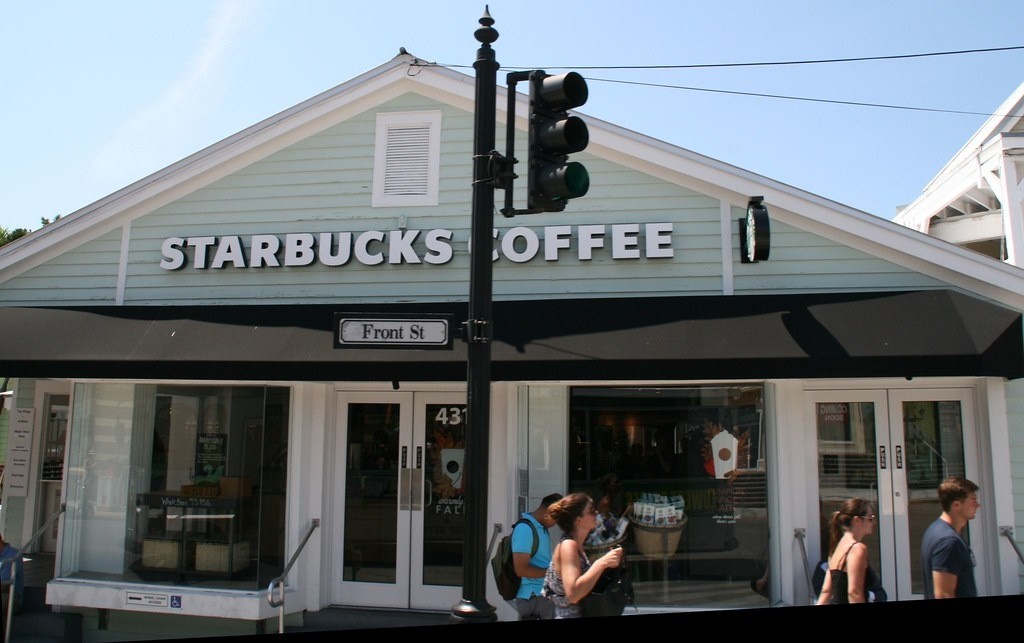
[492, 517, 538, 600]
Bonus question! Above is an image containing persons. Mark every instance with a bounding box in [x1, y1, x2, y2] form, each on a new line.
[350, 410, 775, 593]
[0, 531, 25, 618]
[813, 495, 876, 606]
[540, 492, 624, 619]
[509, 493, 564, 621]
[918, 474, 982, 600]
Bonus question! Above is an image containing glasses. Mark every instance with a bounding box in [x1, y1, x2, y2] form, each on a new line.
[857, 513, 876, 520]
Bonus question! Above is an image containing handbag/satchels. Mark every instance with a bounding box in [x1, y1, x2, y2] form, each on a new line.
[583, 565, 634, 616]
[861, 576, 887, 603]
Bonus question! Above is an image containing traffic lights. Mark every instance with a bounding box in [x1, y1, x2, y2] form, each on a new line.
[521, 66, 589, 216]
